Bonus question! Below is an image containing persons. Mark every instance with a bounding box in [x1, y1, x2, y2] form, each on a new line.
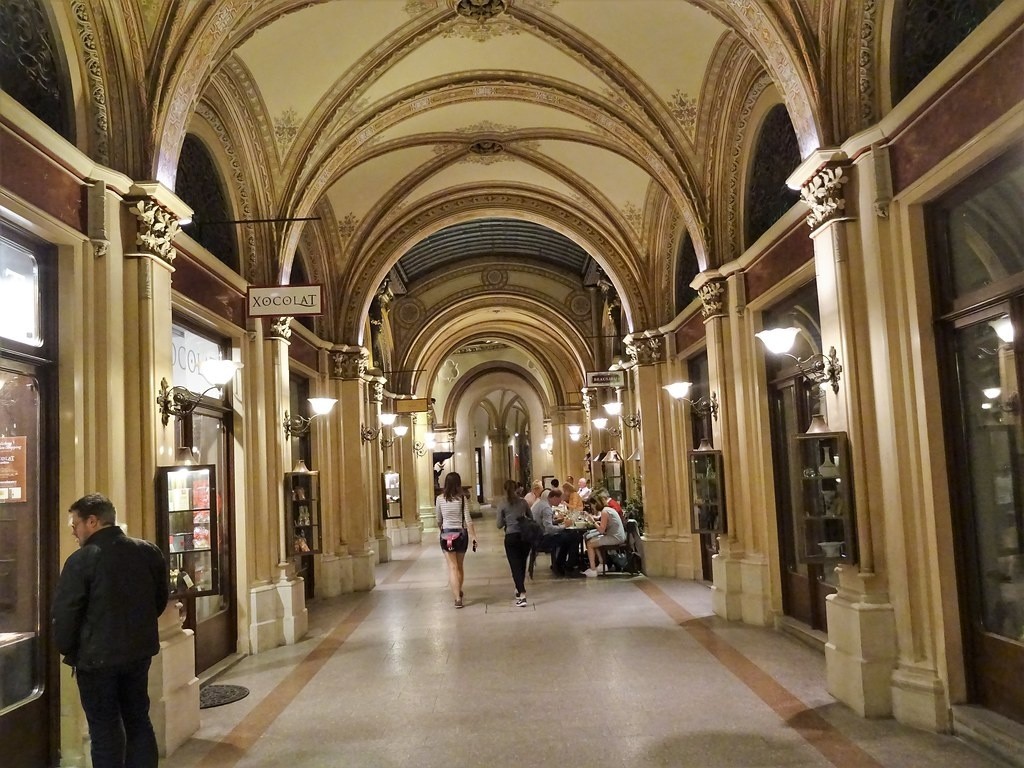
[581, 494, 626, 576]
[54, 492, 167, 768]
[528, 490, 568, 574]
[517, 475, 625, 567]
[498, 480, 533, 607]
[435, 472, 478, 608]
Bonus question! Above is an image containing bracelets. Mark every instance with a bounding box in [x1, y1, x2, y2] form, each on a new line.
[593, 522, 595, 524]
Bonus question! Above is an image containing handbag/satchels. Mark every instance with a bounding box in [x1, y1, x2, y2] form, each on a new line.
[440, 531, 464, 552]
[518, 515, 543, 549]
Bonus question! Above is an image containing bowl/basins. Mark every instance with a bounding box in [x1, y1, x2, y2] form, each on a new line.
[575, 522, 586, 528]
[558, 523, 565, 528]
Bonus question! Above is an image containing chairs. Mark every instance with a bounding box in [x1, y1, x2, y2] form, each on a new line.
[601, 519, 639, 576]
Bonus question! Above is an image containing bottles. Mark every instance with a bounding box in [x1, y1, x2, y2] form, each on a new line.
[818, 446, 837, 477]
[824, 490, 837, 517]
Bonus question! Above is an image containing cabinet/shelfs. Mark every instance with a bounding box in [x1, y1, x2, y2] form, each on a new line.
[155, 448, 220, 600]
[381, 465, 403, 519]
[687, 439, 725, 534]
[788, 431, 856, 566]
[286, 460, 322, 556]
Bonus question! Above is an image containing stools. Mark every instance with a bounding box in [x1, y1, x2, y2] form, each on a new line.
[528, 542, 556, 580]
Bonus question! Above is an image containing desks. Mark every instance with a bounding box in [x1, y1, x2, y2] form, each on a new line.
[552, 526, 590, 576]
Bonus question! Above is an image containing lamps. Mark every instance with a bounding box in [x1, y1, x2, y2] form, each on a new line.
[361, 413, 408, 448]
[568, 425, 590, 448]
[602, 402, 641, 430]
[972, 313, 1014, 356]
[158, 357, 243, 425]
[984, 386, 1021, 417]
[662, 383, 718, 420]
[592, 419, 622, 438]
[414, 433, 436, 458]
[282, 397, 336, 442]
[755, 327, 842, 395]
[540, 438, 552, 455]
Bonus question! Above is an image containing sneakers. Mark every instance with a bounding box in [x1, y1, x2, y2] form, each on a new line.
[581, 569, 598, 578]
[516, 598, 527, 607]
[596, 563, 608, 572]
[455, 598, 463, 608]
[516, 592, 520, 600]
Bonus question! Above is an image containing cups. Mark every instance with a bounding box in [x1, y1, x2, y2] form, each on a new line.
[818, 541, 846, 557]
[172, 533, 184, 550]
[173, 487, 189, 509]
